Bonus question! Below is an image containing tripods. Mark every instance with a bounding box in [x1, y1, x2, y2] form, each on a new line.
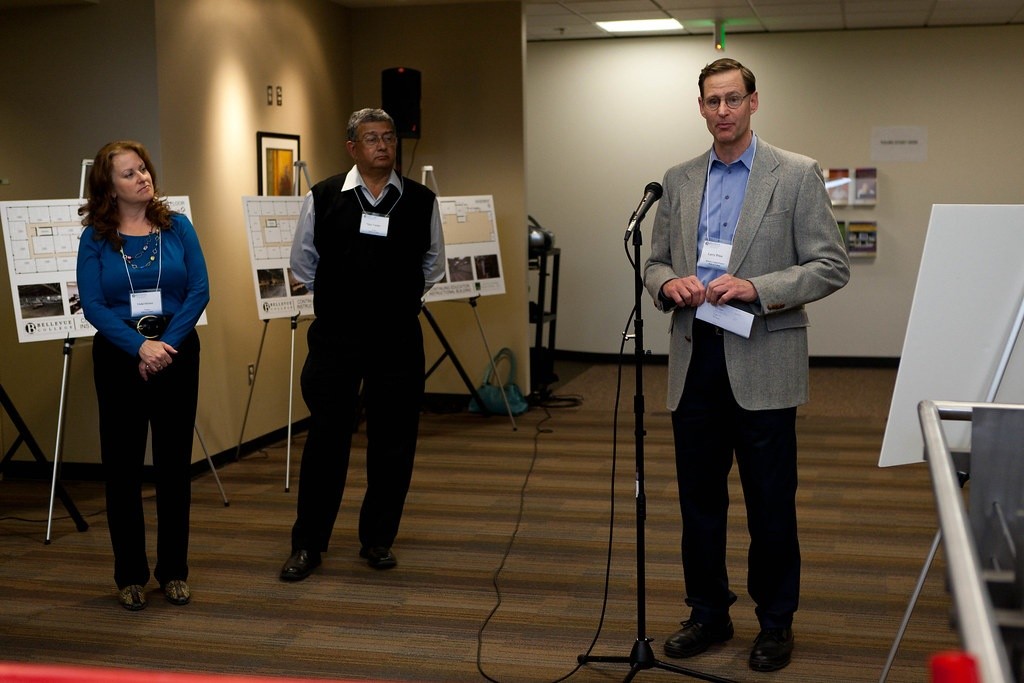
[576, 212, 738, 683]
[352, 138, 490, 435]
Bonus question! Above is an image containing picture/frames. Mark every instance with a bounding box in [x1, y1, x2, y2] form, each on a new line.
[256, 130, 301, 200]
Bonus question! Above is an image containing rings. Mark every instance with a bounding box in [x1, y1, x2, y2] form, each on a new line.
[146, 365, 150, 368]
[156, 365, 161, 368]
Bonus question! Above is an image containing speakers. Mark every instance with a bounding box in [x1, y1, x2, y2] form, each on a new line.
[381, 67, 422, 138]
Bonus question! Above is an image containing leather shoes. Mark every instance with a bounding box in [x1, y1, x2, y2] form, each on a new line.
[748, 629, 795, 673]
[358, 545, 396, 569]
[664, 615, 736, 657]
[280, 547, 321, 582]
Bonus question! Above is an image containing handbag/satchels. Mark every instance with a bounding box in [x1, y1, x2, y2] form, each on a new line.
[468, 348, 529, 414]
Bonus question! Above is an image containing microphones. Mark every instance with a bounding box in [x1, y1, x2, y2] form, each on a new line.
[623, 182, 663, 242]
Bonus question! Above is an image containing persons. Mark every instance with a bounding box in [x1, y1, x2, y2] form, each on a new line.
[858, 182, 874, 198]
[831, 186, 847, 199]
[643, 58, 852, 673]
[280, 108, 449, 579]
[76, 140, 210, 611]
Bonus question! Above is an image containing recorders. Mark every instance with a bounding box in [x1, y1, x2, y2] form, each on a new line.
[528, 216, 556, 257]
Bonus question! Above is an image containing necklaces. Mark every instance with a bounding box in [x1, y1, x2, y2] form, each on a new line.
[119, 225, 159, 269]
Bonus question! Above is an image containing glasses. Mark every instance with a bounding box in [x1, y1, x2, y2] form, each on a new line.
[351, 134, 398, 145]
[702, 89, 756, 112]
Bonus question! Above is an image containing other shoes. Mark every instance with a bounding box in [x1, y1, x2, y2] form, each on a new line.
[121, 585, 147, 611]
[164, 579, 191, 606]
